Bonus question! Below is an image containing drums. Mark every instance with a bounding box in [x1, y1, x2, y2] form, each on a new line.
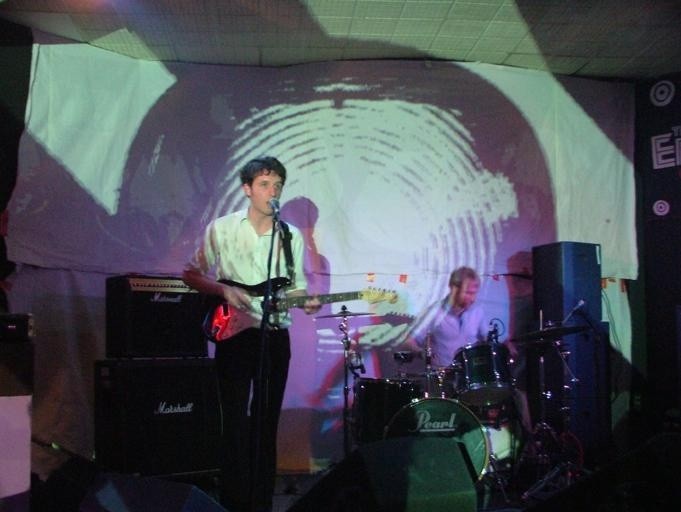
[467, 397, 523, 462]
[382, 399, 489, 491]
[453, 341, 515, 405]
[353, 378, 422, 426]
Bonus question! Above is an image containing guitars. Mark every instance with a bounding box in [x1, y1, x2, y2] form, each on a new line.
[204, 279, 397, 342]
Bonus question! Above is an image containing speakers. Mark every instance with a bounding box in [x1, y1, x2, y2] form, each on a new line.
[532, 242, 610, 448]
[0, 310, 35, 398]
[353, 381, 419, 444]
[72, 468, 222, 512]
[92, 358, 222, 488]
[105, 274, 208, 357]
[286, 436, 489, 512]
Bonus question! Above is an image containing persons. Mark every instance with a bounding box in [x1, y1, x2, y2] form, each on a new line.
[181, 153, 324, 511]
[400, 265, 492, 376]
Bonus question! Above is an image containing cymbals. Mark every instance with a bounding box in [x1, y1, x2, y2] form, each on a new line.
[313, 312, 375, 318]
[510, 323, 599, 342]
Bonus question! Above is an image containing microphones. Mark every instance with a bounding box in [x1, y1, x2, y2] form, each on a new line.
[267, 198, 281, 214]
[522, 460, 568, 500]
[359, 354, 366, 374]
